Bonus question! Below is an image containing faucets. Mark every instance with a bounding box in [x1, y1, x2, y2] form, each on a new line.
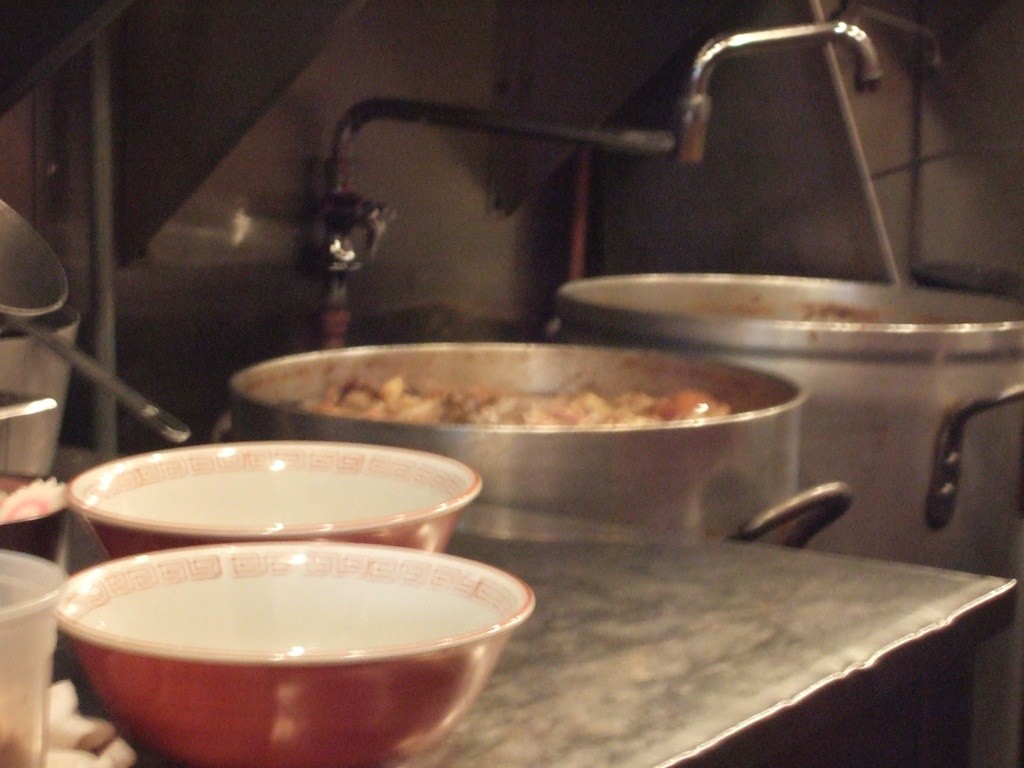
[675, 21, 888, 161]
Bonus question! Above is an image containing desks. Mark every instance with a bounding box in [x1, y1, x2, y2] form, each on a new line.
[52, 446, 1017, 768]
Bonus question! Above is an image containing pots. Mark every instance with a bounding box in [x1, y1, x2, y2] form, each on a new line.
[0, 197, 193, 444]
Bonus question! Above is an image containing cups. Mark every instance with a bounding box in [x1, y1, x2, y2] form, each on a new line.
[0, 549, 69, 768]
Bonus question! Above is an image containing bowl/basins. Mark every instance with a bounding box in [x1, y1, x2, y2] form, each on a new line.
[0, 472, 71, 562]
[56, 538, 536, 768]
[67, 442, 483, 563]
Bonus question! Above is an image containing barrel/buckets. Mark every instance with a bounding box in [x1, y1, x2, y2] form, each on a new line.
[557, 269, 1024, 580]
[0, 303, 80, 478]
[227, 344, 852, 549]
